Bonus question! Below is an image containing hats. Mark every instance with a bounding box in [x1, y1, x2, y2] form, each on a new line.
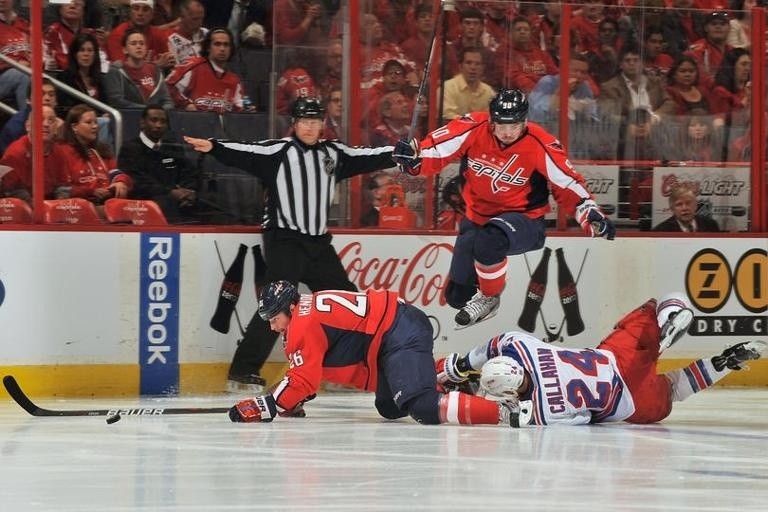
[380, 57, 410, 73]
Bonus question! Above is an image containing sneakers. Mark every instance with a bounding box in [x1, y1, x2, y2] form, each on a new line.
[225, 371, 267, 387]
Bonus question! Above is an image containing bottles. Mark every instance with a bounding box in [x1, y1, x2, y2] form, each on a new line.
[553, 247, 586, 337]
[250, 244, 266, 304]
[210, 244, 248, 335]
[517, 246, 552, 333]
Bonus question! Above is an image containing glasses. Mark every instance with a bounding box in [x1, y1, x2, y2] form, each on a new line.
[384, 70, 403, 75]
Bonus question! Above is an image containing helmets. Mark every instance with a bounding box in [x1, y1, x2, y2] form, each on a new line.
[489, 86, 531, 126]
[255, 278, 301, 326]
[291, 94, 325, 120]
[478, 355, 524, 399]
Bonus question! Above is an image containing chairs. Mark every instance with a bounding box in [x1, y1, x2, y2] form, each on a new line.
[118, 45, 325, 225]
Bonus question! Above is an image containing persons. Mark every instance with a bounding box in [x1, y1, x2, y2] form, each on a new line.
[391, 86, 616, 326]
[183, 94, 419, 386]
[433, 292, 767, 426]
[229, 280, 534, 428]
[0, 0, 768, 232]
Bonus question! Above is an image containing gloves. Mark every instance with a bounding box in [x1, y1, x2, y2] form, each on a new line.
[388, 133, 424, 178]
[575, 198, 617, 243]
[227, 394, 280, 425]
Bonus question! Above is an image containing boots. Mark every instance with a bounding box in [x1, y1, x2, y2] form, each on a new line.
[710, 337, 763, 372]
[658, 306, 694, 348]
[497, 397, 521, 431]
[454, 288, 501, 326]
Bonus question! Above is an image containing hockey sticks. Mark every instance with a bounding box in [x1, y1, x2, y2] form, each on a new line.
[3, 375, 231, 416]
